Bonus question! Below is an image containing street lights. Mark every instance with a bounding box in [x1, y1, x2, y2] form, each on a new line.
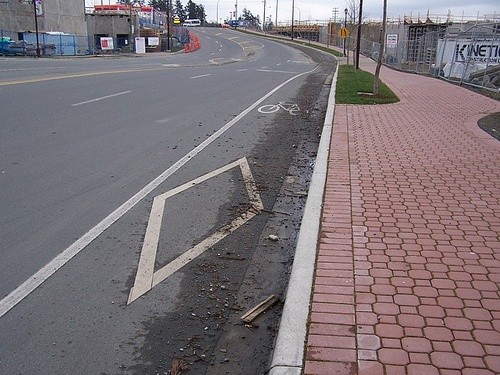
[217, 0, 220, 26]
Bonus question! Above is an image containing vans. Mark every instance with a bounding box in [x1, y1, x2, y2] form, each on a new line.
[184, 19, 201, 27]
